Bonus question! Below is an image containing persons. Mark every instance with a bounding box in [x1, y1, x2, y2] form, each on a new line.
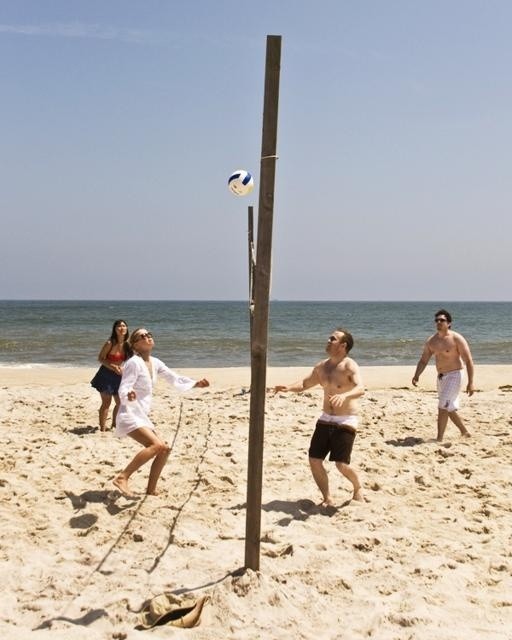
[272, 328, 364, 508]
[88, 320, 134, 432]
[411, 309, 474, 443]
[112, 327, 211, 497]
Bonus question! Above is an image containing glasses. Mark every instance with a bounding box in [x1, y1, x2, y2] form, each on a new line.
[435, 318, 448, 323]
[134, 331, 152, 343]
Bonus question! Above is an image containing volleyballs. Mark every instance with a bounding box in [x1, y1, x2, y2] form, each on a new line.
[228, 170, 254, 197]
